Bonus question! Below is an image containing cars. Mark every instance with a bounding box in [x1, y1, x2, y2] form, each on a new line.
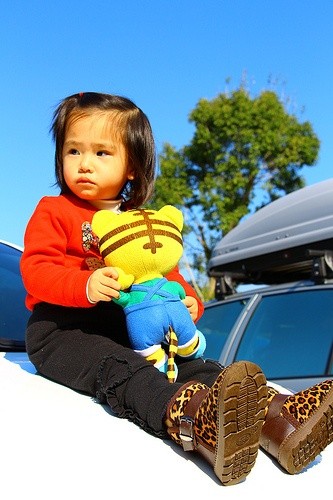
[0, 238, 333, 497]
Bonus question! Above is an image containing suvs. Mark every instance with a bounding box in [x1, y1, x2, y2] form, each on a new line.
[193, 174, 333, 390]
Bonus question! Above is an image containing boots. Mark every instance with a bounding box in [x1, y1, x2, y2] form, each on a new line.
[166, 360, 268, 486]
[259, 378, 333, 475]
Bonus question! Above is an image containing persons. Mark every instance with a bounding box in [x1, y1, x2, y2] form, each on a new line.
[18, 92, 333, 485]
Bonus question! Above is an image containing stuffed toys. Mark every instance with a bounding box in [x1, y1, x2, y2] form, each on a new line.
[93, 206, 208, 382]
